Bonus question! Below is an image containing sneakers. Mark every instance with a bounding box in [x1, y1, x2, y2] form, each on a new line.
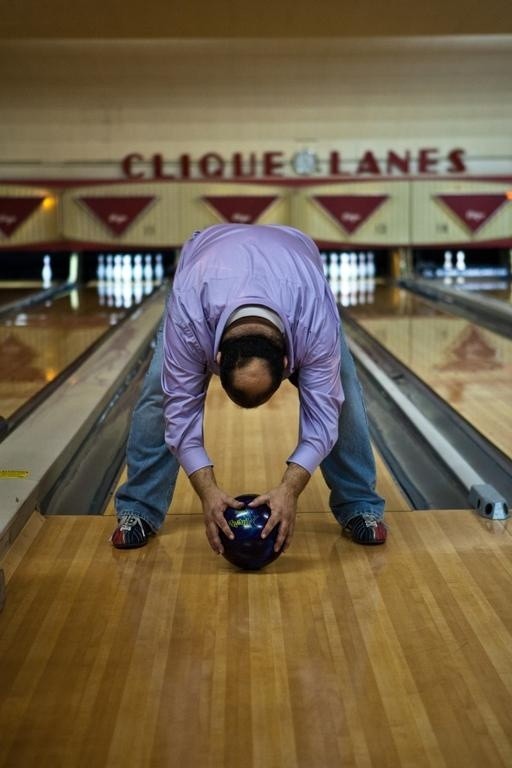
[111, 515, 153, 548]
[342, 514, 386, 544]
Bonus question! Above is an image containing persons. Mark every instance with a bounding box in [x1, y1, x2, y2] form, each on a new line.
[111, 222, 388, 549]
